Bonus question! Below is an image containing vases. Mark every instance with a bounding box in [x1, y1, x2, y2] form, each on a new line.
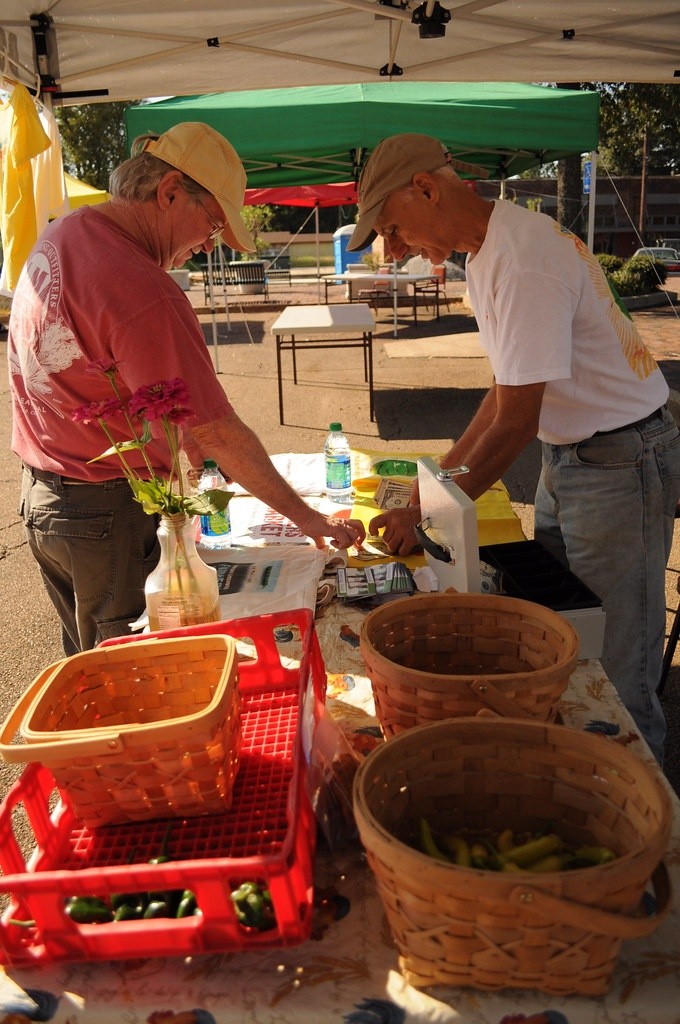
[143, 511, 220, 633]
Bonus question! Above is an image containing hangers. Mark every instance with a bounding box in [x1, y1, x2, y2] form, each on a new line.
[1, 45, 44, 111]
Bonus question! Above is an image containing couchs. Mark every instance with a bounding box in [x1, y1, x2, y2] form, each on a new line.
[345, 264, 410, 299]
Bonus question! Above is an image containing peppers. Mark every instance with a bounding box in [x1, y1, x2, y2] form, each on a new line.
[412, 816, 621, 874]
[8, 823, 278, 932]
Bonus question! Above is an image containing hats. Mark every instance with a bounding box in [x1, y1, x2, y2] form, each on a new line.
[141, 122, 258, 253]
[344, 134, 490, 252]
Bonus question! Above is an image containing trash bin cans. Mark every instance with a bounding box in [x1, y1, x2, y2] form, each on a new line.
[333, 224, 373, 284]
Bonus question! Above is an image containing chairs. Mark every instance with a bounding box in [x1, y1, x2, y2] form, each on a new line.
[359, 266, 392, 312]
[410, 264, 450, 315]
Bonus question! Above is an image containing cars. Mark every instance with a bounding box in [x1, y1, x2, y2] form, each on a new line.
[631, 238, 680, 272]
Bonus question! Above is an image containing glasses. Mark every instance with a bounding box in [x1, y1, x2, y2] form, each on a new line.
[197, 198, 225, 240]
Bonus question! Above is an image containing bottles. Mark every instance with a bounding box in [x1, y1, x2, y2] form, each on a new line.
[323, 422, 353, 504]
[197, 457, 233, 551]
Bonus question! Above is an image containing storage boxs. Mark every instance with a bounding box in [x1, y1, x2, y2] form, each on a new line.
[0, 609, 328, 967]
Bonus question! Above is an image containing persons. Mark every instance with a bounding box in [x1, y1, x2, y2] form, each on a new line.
[6, 123, 366, 659]
[342, 132, 680, 768]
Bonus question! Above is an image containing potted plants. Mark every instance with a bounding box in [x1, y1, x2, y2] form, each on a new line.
[228, 203, 272, 296]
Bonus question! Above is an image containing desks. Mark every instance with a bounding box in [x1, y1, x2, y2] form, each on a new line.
[322, 273, 441, 329]
[0, 454, 680, 1024]
[272, 304, 376, 424]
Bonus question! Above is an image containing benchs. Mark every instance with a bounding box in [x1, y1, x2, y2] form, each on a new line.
[200, 260, 272, 302]
[256, 255, 291, 288]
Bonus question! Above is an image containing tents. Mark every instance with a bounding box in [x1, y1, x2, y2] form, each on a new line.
[0, 0, 680, 376]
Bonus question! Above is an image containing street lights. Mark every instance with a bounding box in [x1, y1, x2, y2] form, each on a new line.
[500, 174, 522, 201]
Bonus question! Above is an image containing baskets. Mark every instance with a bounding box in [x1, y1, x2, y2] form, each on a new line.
[354, 717, 676, 996]
[359, 587, 582, 741]
[0, 635, 245, 827]
[0, 606, 328, 968]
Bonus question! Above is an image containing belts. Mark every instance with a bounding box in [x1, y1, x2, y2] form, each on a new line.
[591, 404, 668, 437]
[31, 467, 128, 486]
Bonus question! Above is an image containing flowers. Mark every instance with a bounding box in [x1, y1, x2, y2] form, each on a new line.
[71, 358, 235, 619]
